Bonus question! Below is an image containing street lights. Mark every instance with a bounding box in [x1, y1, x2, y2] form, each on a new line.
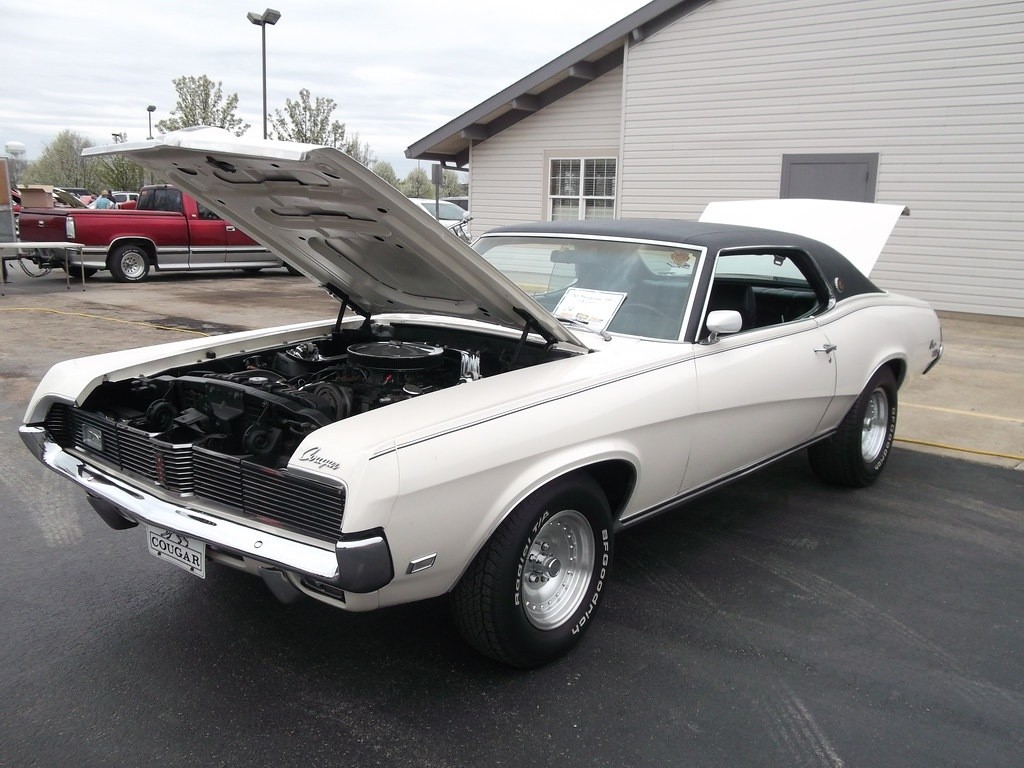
[147, 105, 157, 185]
[245, 8, 283, 140]
[111, 133, 120, 191]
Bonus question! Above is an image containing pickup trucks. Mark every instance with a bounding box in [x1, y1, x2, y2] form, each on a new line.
[18, 183, 305, 286]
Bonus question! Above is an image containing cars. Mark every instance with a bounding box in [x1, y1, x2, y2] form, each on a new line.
[10, 183, 141, 238]
[409, 196, 468, 237]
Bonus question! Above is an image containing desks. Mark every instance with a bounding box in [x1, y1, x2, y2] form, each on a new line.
[0, 242, 86, 296]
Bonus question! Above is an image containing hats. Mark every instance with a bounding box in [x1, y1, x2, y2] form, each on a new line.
[102, 190, 110, 195]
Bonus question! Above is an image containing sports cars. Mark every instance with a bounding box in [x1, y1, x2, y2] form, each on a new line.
[19, 126, 946, 672]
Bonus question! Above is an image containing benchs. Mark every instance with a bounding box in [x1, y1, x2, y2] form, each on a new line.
[632, 280, 814, 331]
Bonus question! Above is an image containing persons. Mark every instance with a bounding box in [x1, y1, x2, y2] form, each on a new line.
[87, 189, 116, 209]
